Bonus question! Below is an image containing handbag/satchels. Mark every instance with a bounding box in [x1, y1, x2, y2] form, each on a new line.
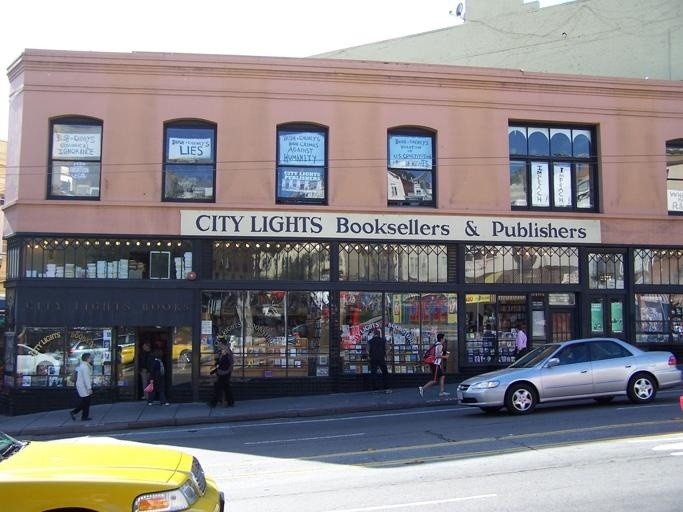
[70, 369, 78, 382]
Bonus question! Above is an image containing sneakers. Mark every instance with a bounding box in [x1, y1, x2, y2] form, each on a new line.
[224, 401, 235, 408]
[439, 391, 450, 397]
[418, 386, 425, 399]
[370, 390, 379, 395]
[206, 401, 216, 408]
[141, 395, 154, 406]
[384, 389, 393, 394]
[70, 411, 92, 422]
[159, 402, 170, 407]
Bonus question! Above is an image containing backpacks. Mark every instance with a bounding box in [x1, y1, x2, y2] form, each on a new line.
[423, 341, 441, 364]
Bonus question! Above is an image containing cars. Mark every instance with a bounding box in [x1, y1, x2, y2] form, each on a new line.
[0, 427, 227, 512]
[454, 337, 682, 416]
[14, 330, 215, 377]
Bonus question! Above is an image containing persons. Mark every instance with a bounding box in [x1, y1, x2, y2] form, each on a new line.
[207, 339, 234, 408]
[116, 346, 123, 381]
[514, 325, 527, 361]
[138, 340, 170, 406]
[417, 334, 450, 398]
[365, 330, 392, 395]
[482, 324, 496, 362]
[69, 353, 93, 421]
